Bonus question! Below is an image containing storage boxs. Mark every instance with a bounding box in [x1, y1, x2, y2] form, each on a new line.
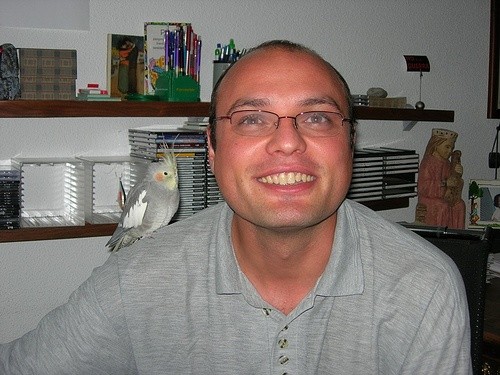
[19, 48, 78, 100]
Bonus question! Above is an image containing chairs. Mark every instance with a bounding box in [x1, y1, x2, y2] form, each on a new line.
[425, 238, 490, 375]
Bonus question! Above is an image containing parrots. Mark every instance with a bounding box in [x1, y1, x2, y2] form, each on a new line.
[99, 133, 184, 253]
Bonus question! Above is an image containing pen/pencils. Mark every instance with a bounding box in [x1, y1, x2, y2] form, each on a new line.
[165, 24, 202, 83]
[215, 38, 246, 62]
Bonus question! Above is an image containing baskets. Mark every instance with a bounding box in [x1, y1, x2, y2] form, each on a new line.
[369, 95, 406, 107]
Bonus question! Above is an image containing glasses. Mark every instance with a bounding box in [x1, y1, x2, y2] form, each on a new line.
[213, 109, 358, 137]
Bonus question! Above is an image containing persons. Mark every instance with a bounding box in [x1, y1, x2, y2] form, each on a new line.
[416, 127, 466, 231]
[0, 39, 472, 374]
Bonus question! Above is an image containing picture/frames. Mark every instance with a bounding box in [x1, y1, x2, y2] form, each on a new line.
[465, 178, 500, 230]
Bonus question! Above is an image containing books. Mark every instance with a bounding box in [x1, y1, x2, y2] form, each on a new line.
[348, 146, 419, 202]
[128, 125, 225, 224]
[107, 33, 146, 98]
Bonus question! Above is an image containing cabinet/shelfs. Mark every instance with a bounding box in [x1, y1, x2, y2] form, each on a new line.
[0, 100, 454, 242]
